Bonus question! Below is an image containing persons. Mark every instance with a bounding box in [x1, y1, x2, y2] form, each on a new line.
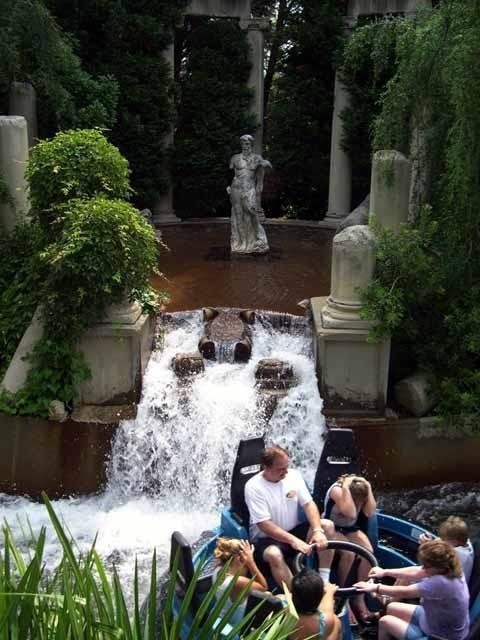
[226, 133, 270, 257]
[197, 443, 476, 640]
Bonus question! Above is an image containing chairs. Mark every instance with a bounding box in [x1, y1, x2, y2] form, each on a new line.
[170, 531, 219, 625]
[314, 428, 379, 554]
[222, 438, 268, 542]
[467, 541, 480, 625]
